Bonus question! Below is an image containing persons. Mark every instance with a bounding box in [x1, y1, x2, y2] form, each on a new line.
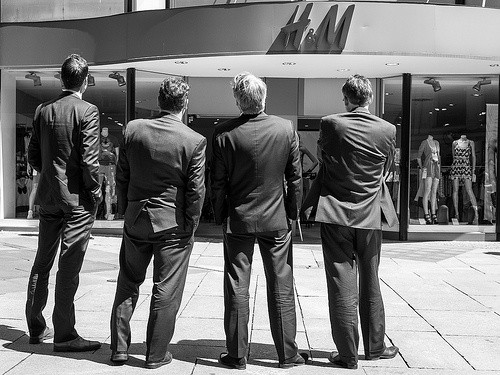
[296, 132, 317, 233]
[24, 53, 101, 352]
[450, 134, 480, 224]
[416, 134, 442, 225]
[97, 127, 119, 219]
[211, 70, 311, 371]
[110, 74, 207, 369]
[313, 74, 402, 369]
[27, 160, 42, 219]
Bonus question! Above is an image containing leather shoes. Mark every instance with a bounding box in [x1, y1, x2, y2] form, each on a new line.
[219, 352, 247, 370]
[328, 351, 358, 369]
[29, 326, 54, 344]
[278, 352, 308, 369]
[145, 352, 172, 369]
[111, 349, 128, 362]
[365, 345, 399, 361]
[53, 337, 100, 353]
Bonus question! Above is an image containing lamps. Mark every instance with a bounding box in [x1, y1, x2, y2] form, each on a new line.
[473, 78, 491, 92]
[25, 71, 126, 87]
[423, 77, 441, 92]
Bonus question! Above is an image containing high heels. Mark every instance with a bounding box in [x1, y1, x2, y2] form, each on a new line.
[431, 214, 438, 224]
[424, 214, 432, 224]
[27, 210, 33, 219]
